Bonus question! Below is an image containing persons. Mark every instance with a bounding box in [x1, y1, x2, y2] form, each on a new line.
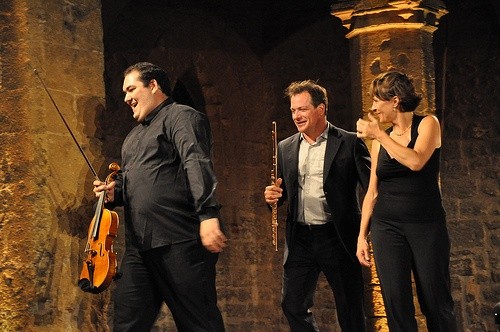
[356, 71, 457, 332]
[264, 80, 372, 332]
[93, 62, 228, 332]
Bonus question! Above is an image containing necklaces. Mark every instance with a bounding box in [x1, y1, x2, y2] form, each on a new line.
[393, 125, 411, 136]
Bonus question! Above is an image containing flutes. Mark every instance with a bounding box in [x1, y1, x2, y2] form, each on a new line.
[271, 120, 278, 252]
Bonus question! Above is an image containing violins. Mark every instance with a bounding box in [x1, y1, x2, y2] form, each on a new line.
[77, 162, 120, 293]
